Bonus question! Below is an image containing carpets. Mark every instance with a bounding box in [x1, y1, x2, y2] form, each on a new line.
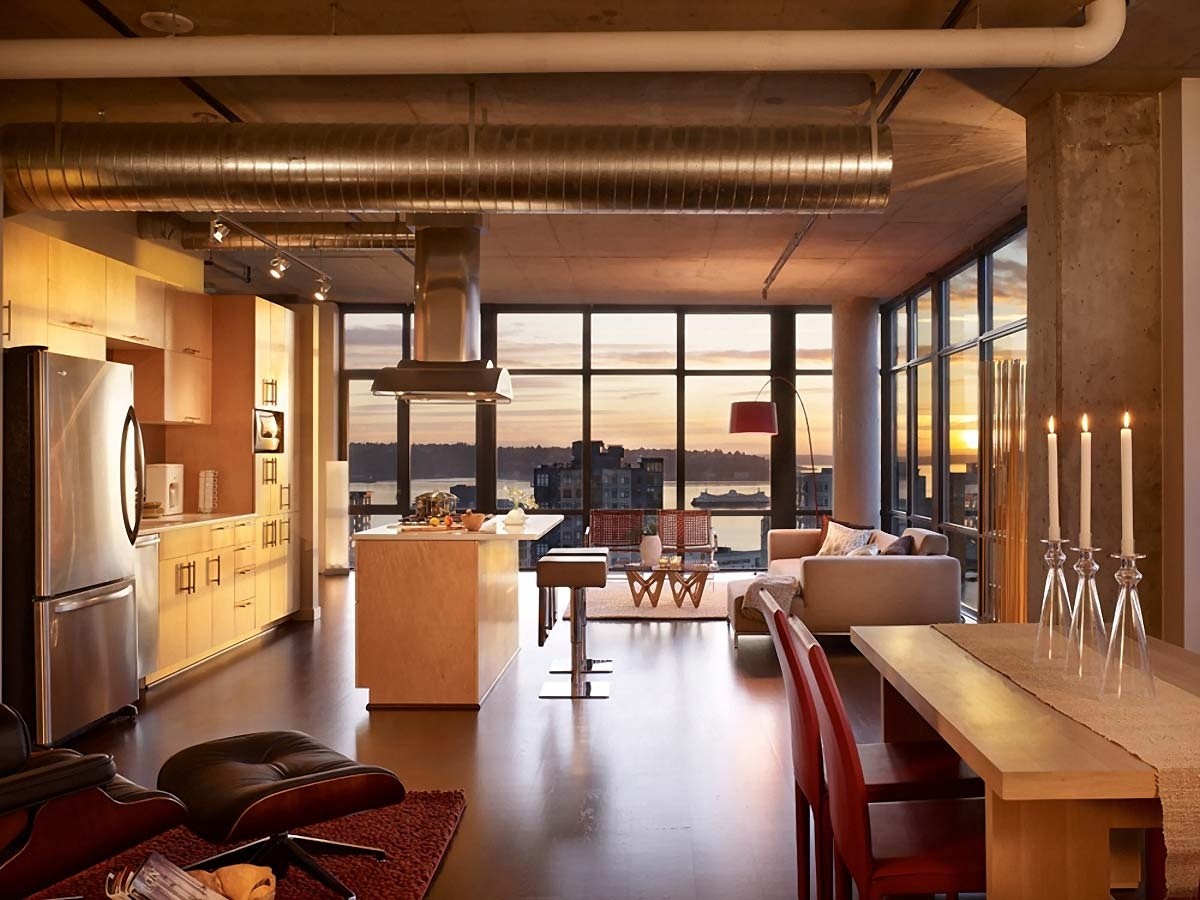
[12, 787, 469, 900]
[564, 571, 732, 621]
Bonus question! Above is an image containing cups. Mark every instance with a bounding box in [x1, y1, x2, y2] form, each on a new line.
[198, 469, 218, 513]
[462, 513, 486, 531]
[657, 555, 682, 568]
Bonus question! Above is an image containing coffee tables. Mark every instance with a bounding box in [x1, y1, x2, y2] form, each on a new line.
[607, 559, 719, 608]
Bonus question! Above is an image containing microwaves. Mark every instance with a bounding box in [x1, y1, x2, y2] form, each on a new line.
[254, 409, 285, 452]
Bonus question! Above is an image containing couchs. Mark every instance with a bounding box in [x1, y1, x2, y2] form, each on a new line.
[0, 701, 187, 899]
[768, 526, 962, 650]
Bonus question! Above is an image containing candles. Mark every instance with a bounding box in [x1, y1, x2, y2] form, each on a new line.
[1047, 431, 1062, 541]
[1079, 431, 1093, 549]
[1121, 428, 1136, 557]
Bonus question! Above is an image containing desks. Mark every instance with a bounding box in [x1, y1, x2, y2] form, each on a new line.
[354, 512, 565, 711]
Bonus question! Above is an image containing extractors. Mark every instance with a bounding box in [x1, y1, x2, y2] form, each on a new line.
[370, 212, 512, 405]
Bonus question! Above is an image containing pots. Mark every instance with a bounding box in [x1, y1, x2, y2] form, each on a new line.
[417, 489, 458, 521]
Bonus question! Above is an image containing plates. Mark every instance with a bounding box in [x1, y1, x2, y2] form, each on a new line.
[144, 502, 163, 519]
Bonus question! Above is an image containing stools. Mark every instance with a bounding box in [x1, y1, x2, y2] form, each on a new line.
[544, 547, 613, 674]
[536, 556, 613, 699]
[156, 730, 406, 900]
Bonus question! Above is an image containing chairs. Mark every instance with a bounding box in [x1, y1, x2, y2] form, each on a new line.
[785, 611, 986, 900]
[583, 509, 645, 581]
[655, 508, 719, 592]
[757, 588, 985, 900]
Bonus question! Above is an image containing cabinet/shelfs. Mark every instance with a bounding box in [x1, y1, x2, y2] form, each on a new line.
[0, 219, 213, 427]
[136, 294, 302, 694]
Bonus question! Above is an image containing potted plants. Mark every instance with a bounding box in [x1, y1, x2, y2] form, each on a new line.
[639, 523, 664, 565]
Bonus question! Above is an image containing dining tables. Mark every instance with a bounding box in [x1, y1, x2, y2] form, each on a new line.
[849, 622, 1199, 900]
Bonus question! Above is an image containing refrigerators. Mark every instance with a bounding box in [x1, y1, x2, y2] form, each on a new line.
[1, 343, 145, 749]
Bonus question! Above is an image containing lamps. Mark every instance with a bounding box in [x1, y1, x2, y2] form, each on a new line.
[315, 277, 334, 300]
[215, 221, 230, 243]
[729, 376, 821, 529]
[271, 256, 292, 280]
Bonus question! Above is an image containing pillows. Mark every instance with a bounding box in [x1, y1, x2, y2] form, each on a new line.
[824, 516, 875, 545]
[816, 523, 872, 558]
[845, 542, 879, 557]
[877, 534, 913, 556]
